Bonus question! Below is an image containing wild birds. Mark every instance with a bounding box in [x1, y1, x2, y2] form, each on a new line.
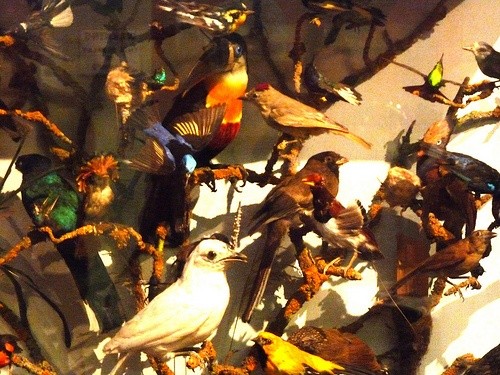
[1, 0, 500, 375]
